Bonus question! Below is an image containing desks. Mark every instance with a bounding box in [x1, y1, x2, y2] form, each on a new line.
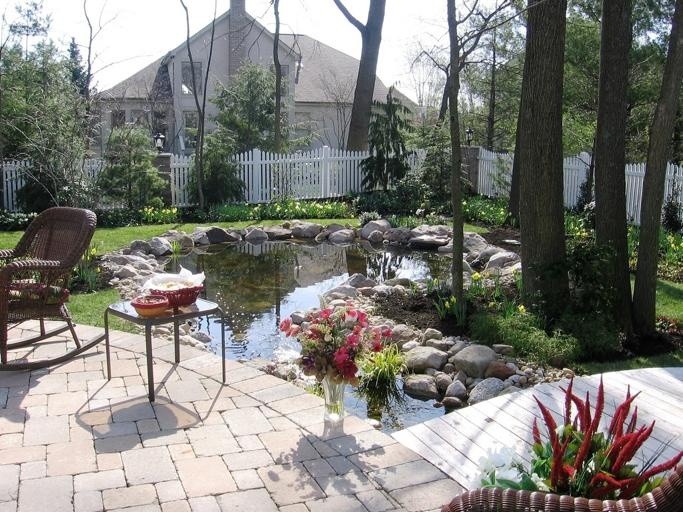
[103, 296, 226, 403]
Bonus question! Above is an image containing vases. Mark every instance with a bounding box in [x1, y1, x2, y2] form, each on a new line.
[321, 373, 346, 438]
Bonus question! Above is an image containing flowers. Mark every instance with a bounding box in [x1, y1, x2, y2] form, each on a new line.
[279, 292, 393, 415]
[478, 371, 683, 499]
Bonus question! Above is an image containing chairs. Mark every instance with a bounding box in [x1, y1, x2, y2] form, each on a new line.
[0, 206, 107, 371]
[440, 458, 682, 511]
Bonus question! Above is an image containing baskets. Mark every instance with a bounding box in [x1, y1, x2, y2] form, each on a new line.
[149, 283, 204, 307]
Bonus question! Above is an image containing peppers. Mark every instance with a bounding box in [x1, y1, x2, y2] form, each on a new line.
[531, 372, 683, 499]
